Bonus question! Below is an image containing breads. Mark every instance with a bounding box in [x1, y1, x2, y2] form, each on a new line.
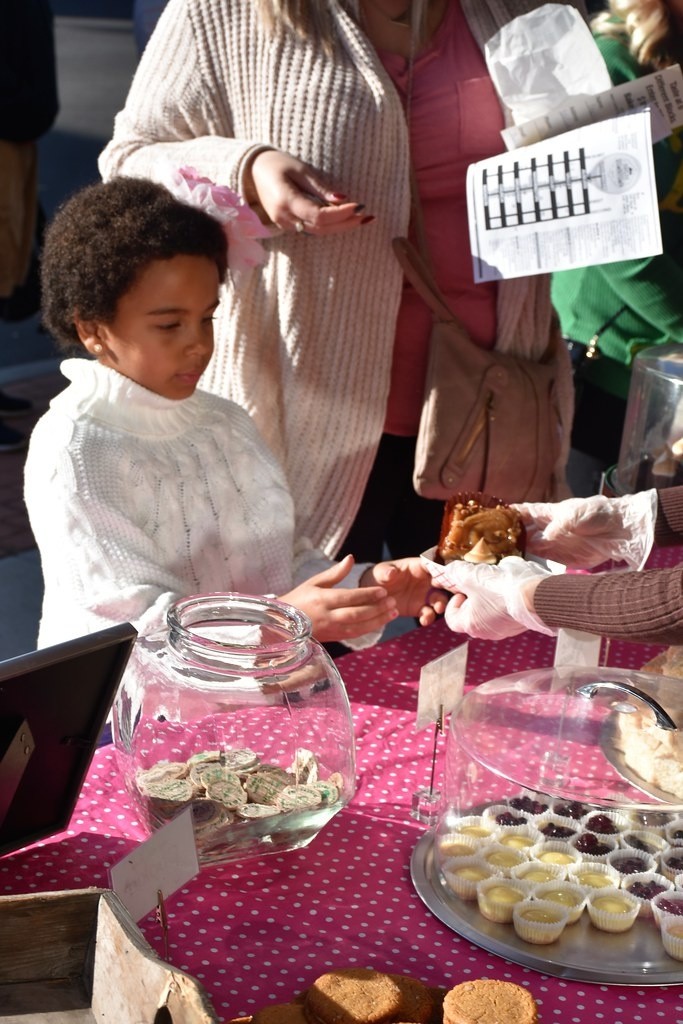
[617, 644, 683, 803]
[225, 967, 539, 1024]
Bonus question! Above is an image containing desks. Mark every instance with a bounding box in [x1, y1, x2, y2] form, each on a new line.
[0, 545, 683, 1024]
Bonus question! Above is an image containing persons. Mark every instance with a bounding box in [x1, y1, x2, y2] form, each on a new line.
[24, 175, 446, 743]
[100, 0, 590, 661]
[431, 486, 683, 641]
[550, 0, 683, 496]
[0, 0, 59, 452]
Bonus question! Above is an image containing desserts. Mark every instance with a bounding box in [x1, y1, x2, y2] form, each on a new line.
[437, 788, 682, 963]
[435, 490, 527, 566]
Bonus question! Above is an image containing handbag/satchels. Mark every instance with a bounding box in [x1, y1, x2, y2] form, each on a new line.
[564, 337, 630, 463]
[391, 235, 560, 506]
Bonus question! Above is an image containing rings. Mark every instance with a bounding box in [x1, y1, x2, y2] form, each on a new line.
[294, 219, 305, 233]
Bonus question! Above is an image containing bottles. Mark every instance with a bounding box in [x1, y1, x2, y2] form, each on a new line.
[111, 592, 358, 868]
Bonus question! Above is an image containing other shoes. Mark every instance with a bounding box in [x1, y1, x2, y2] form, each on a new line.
[0, 422, 27, 451]
[0, 392, 32, 416]
